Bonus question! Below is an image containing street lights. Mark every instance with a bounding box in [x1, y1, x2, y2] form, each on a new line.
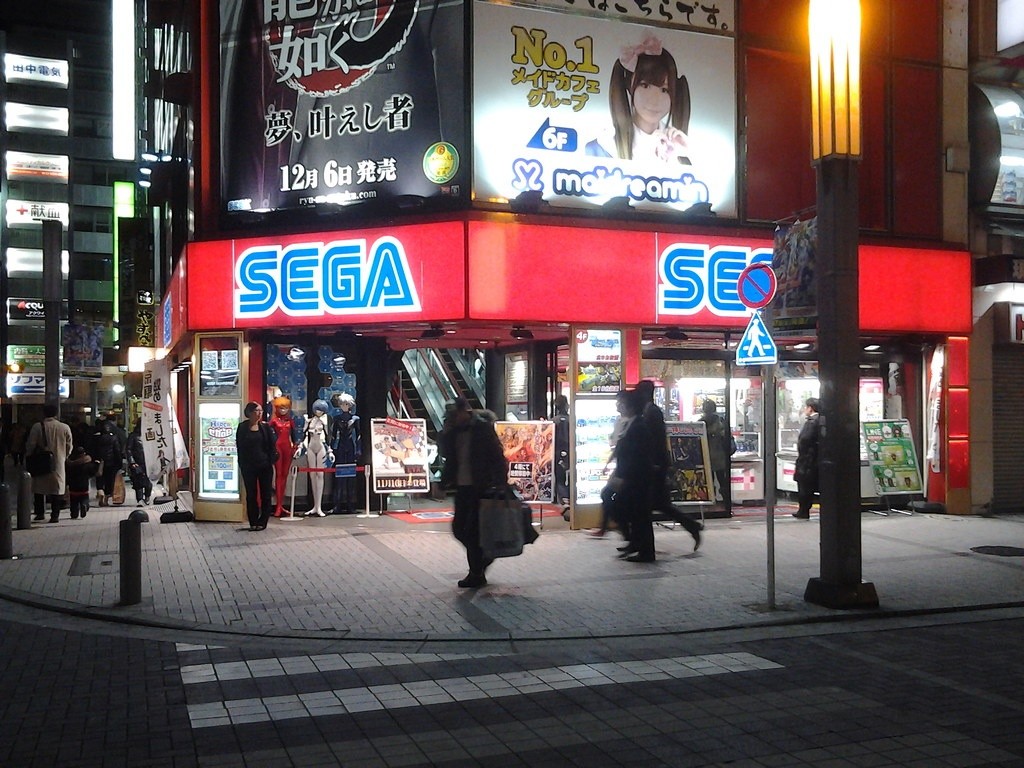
[113, 384, 130, 476]
[803, 0, 882, 607]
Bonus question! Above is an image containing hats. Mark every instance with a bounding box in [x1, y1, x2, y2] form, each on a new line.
[443, 397, 465, 416]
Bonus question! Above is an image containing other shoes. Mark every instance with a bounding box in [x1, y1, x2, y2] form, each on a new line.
[792, 511, 810, 519]
[617, 545, 638, 551]
[249, 522, 257, 531]
[590, 529, 605, 537]
[691, 520, 705, 551]
[49, 518, 58, 523]
[80, 504, 86, 517]
[458, 568, 487, 588]
[34, 515, 44, 520]
[136, 500, 143, 507]
[257, 521, 266, 530]
[146, 495, 150, 505]
[98, 489, 104, 506]
[626, 553, 655, 562]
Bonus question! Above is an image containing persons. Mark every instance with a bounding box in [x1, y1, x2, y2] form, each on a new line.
[26, 406, 73, 523]
[65, 414, 126, 519]
[697, 399, 737, 493]
[236, 402, 274, 531]
[548, 395, 570, 515]
[792, 398, 819, 519]
[584, 380, 704, 561]
[0, 418, 30, 466]
[437, 396, 509, 586]
[267, 396, 298, 516]
[329, 392, 361, 514]
[126, 417, 153, 507]
[608, 34, 691, 166]
[293, 399, 335, 516]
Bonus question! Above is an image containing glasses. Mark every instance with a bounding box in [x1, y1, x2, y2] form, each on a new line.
[254, 410, 264, 412]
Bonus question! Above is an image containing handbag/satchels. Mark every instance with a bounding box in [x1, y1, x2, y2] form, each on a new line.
[112, 472, 125, 504]
[26, 451, 54, 477]
[478, 490, 538, 557]
